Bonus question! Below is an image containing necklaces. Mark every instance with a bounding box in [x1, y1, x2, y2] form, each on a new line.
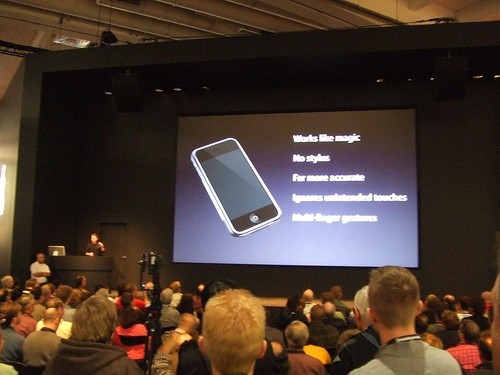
[385, 334, 422, 344]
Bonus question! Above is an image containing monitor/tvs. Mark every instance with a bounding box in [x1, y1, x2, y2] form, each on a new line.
[48, 246, 65, 256]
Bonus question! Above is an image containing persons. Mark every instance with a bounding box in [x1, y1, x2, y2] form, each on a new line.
[347, 265, 463, 375]
[84, 232, 107, 257]
[0, 232, 500, 375]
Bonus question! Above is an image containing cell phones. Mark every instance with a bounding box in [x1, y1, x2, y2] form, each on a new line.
[191, 138, 282, 237]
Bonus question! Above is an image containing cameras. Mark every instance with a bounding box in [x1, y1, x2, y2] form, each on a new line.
[138, 252, 163, 274]
